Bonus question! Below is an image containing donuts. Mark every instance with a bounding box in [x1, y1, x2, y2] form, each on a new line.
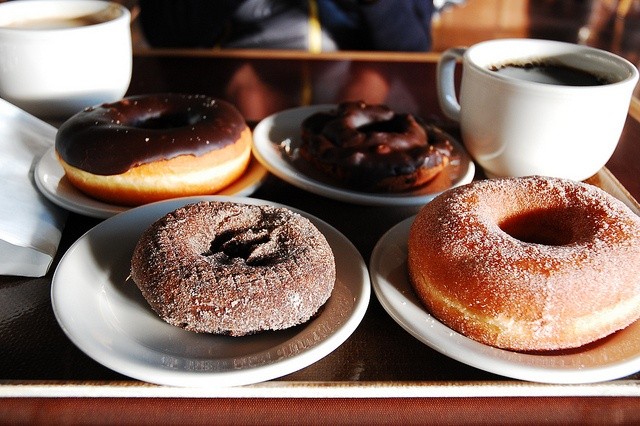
[300, 100, 454, 190]
[130, 200, 337, 336]
[408, 175, 639, 351]
[54, 94, 252, 206]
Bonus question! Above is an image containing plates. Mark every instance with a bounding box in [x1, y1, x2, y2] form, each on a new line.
[33, 144, 269, 220]
[50, 194, 371, 387]
[252, 103, 476, 206]
[369, 213, 640, 384]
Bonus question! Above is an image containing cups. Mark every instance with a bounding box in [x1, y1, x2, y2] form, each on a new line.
[436, 38, 640, 183]
[0, 0, 135, 126]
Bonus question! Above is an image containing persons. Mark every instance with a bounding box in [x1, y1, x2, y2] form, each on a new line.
[140, 0, 433, 57]
